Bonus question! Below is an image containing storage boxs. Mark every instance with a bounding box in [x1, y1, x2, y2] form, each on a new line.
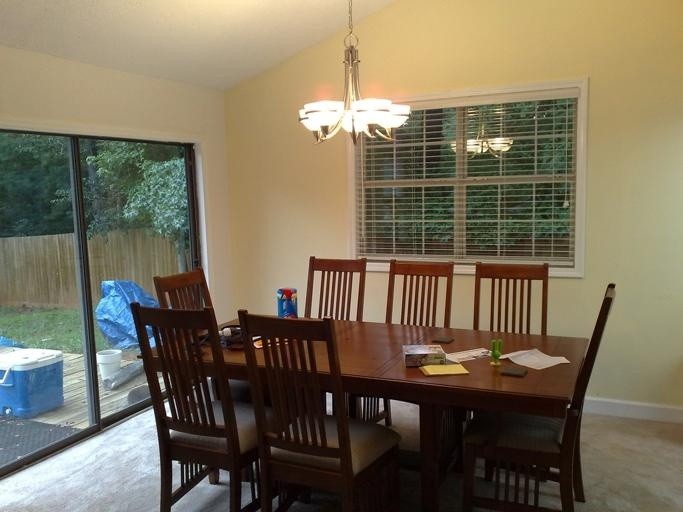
[1, 347, 62, 416]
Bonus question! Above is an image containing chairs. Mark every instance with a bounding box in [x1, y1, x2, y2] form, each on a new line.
[131, 302, 257, 512]
[473, 261, 549, 337]
[478, 283, 616, 511]
[385, 257, 454, 327]
[303, 256, 367, 321]
[154, 266, 219, 340]
[236, 307, 402, 511]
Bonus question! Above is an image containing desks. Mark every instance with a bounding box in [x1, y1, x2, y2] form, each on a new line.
[138, 313, 590, 508]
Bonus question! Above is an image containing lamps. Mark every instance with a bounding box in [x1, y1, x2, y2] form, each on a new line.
[298, 2, 413, 147]
[449, 105, 515, 157]
